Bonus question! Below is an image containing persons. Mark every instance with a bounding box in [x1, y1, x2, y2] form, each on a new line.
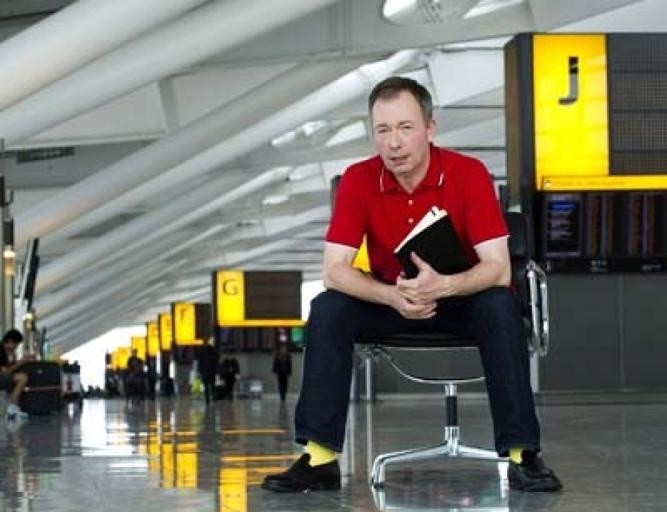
[102, 335, 241, 409]
[0, 425, 37, 512]
[125, 411, 145, 454]
[269, 342, 294, 401]
[258, 76, 564, 498]
[0, 327, 36, 421]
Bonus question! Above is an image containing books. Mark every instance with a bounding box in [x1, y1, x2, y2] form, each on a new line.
[390, 203, 482, 317]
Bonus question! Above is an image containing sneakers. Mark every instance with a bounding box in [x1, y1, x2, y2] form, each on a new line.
[4, 404, 29, 420]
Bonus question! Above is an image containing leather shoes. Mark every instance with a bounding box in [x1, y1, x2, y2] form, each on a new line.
[505, 449, 563, 494]
[260, 453, 343, 494]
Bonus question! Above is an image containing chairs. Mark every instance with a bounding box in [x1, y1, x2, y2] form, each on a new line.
[363, 209, 552, 494]
